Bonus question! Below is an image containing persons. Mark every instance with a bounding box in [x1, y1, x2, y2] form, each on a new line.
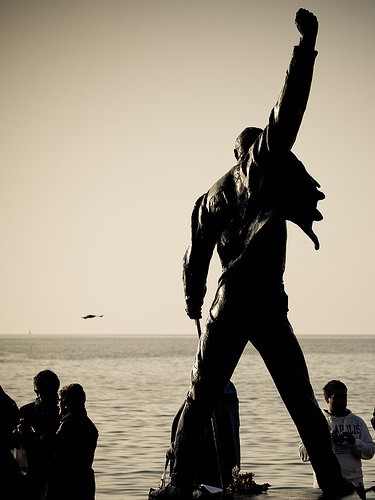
[0, 369, 98, 500]
[298, 380, 375, 500]
[149, 8, 357, 500]
